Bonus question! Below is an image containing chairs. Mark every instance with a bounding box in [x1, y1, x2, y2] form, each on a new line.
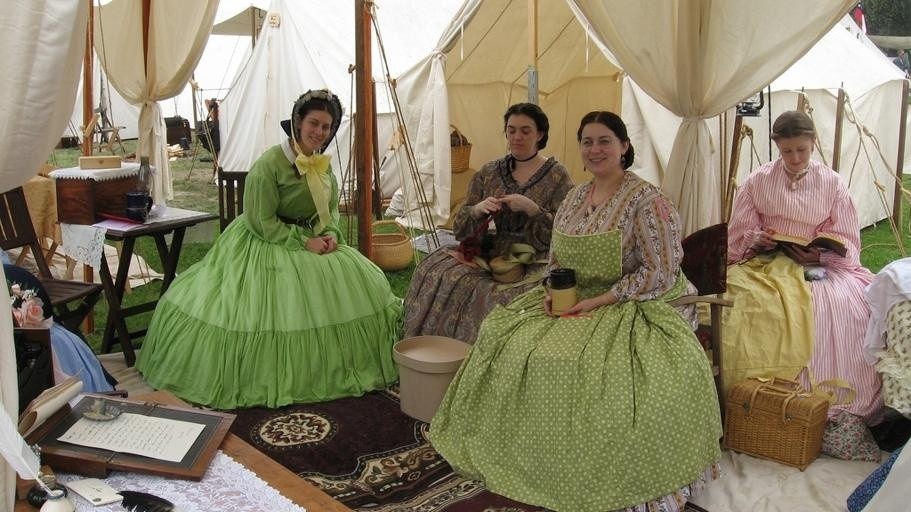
[1, 187, 118, 385]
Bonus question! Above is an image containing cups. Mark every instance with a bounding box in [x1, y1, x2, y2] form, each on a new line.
[541, 268, 578, 316]
[125, 191, 154, 223]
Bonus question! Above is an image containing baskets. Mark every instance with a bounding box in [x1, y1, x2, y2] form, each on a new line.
[723, 376, 828, 472]
[451, 124, 472, 173]
[370, 220, 414, 272]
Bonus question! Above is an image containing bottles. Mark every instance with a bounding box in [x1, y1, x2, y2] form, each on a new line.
[136, 155, 156, 218]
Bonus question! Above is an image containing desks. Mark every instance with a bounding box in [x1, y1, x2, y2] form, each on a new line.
[10, 390, 355, 510]
[54, 207, 218, 369]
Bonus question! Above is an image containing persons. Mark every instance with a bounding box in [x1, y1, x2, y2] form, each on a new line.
[196, 97, 220, 152]
[401, 101, 577, 343]
[2, 249, 116, 394]
[135, 88, 406, 411]
[431, 110, 726, 511]
[727, 108, 884, 419]
[861, 249, 911, 422]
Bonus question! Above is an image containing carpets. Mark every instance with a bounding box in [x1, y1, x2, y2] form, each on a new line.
[229, 387, 706, 511]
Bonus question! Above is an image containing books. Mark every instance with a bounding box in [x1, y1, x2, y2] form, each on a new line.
[17, 365, 82, 426]
[773, 231, 847, 259]
[93, 211, 151, 234]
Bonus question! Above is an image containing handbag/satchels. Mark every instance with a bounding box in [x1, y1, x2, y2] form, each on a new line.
[821, 411, 882, 463]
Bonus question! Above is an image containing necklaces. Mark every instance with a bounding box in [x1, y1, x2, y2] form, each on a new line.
[508, 152, 538, 171]
